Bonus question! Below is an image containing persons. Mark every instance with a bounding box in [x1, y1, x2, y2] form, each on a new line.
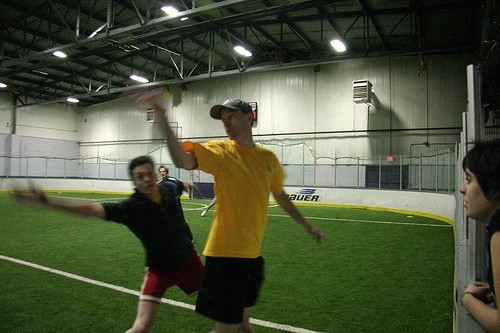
[156, 165, 178, 185]
[12, 156, 205, 333]
[152, 100, 327, 333]
[458, 138, 500, 333]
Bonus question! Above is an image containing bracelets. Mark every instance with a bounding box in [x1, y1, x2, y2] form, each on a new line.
[460, 291, 475, 307]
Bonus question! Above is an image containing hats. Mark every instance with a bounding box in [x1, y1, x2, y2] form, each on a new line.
[210, 100, 252, 120]
